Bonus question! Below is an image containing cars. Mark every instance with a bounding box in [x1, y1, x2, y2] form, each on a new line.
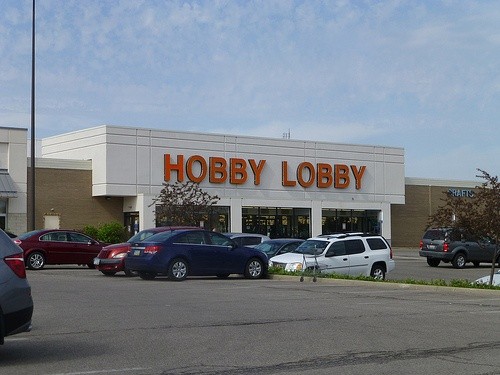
[124, 228, 268, 282]
[211, 231, 271, 248]
[0, 226, 34, 345]
[254, 238, 308, 259]
[11, 227, 108, 271]
[93, 225, 218, 278]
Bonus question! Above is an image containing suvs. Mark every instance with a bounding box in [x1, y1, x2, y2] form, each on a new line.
[418, 226, 500, 269]
[269, 230, 395, 281]
